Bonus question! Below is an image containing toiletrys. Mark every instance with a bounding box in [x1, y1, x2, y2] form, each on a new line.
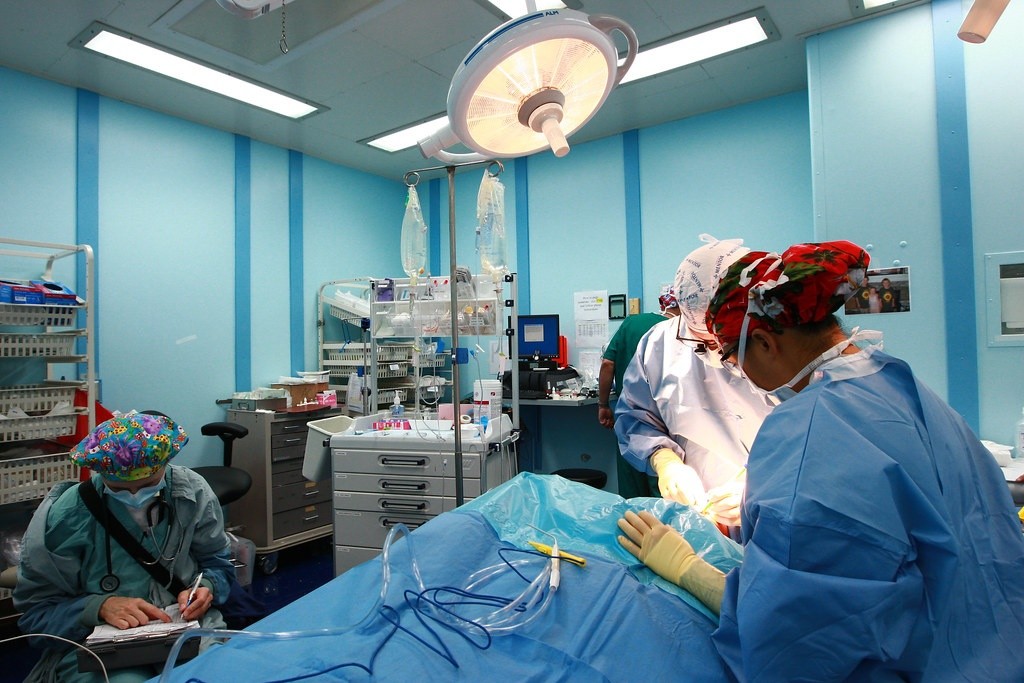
[389, 390, 404, 419]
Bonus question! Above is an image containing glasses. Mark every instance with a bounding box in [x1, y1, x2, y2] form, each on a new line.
[676, 311, 717, 348]
[719, 329, 784, 376]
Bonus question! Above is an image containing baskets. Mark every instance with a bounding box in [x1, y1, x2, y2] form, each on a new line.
[325, 305, 449, 405]
[1, 384, 79, 413]
[0, 332, 77, 360]
[0, 300, 77, 327]
[0, 452, 81, 506]
[1, 413, 78, 444]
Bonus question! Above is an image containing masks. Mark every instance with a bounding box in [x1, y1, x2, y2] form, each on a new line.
[102, 471, 167, 510]
[693, 345, 724, 369]
[741, 370, 798, 408]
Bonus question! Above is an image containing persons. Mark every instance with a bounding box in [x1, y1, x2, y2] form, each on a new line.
[598, 287, 681, 500]
[13, 411, 234, 682]
[856, 276, 870, 314]
[618, 241, 1024, 683]
[613, 235, 797, 542]
[869, 286, 882, 313]
[879, 277, 896, 312]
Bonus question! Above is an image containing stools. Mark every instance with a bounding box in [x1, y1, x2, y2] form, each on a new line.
[551, 468, 609, 490]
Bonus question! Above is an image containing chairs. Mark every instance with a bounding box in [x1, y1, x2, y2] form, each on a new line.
[142, 410, 253, 532]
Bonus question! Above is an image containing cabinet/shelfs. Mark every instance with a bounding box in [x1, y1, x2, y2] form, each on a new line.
[228, 271, 521, 577]
[0, 238, 96, 634]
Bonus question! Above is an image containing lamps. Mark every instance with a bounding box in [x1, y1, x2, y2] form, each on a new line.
[417, 0, 640, 164]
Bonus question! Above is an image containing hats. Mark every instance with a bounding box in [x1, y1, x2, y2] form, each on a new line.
[705, 241, 871, 348]
[68, 414, 189, 483]
[659, 286, 679, 311]
[674, 234, 752, 331]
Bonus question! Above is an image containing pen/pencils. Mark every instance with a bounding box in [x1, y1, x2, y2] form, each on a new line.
[180, 572, 204, 620]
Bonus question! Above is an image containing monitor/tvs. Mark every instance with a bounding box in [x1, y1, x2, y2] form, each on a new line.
[508, 315, 559, 363]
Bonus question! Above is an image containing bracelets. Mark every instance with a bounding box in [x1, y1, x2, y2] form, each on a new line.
[599, 403, 609, 407]
